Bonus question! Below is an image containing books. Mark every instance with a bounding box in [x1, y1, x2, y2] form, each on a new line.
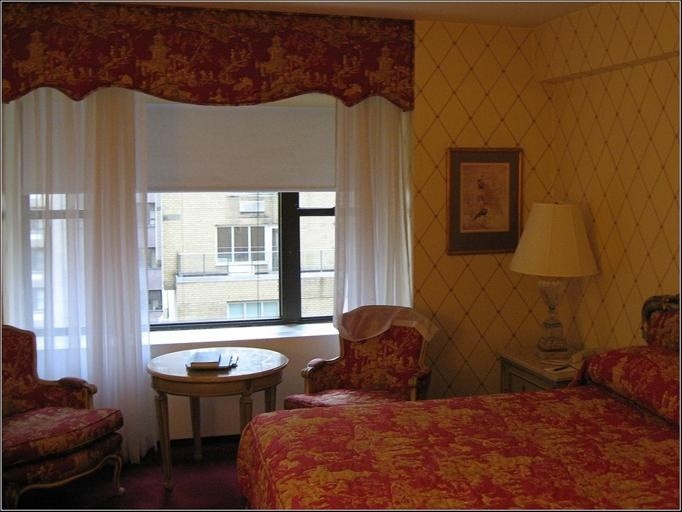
[185, 350, 238, 370]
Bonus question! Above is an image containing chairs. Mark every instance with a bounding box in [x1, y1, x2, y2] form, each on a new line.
[283, 306, 437, 409]
[2, 326, 128, 509]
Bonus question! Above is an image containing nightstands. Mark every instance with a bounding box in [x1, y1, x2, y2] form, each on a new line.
[500, 355, 581, 391]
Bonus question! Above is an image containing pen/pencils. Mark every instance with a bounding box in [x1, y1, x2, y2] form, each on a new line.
[554, 366, 568, 371]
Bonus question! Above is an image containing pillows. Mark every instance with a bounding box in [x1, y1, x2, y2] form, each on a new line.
[582, 344, 679, 427]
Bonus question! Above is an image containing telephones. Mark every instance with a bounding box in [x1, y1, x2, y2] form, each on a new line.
[569, 349, 594, 369]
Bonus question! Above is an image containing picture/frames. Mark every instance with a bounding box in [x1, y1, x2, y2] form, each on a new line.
[445, 147, 521, 257]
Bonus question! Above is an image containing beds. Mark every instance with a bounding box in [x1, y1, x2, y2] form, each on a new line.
[236, 295, 680, 509]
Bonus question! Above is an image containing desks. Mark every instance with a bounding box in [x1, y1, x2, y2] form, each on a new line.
[146, 347, 289, 502]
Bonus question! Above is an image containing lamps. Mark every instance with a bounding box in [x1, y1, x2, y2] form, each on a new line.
[508, 202, 599, 356]
